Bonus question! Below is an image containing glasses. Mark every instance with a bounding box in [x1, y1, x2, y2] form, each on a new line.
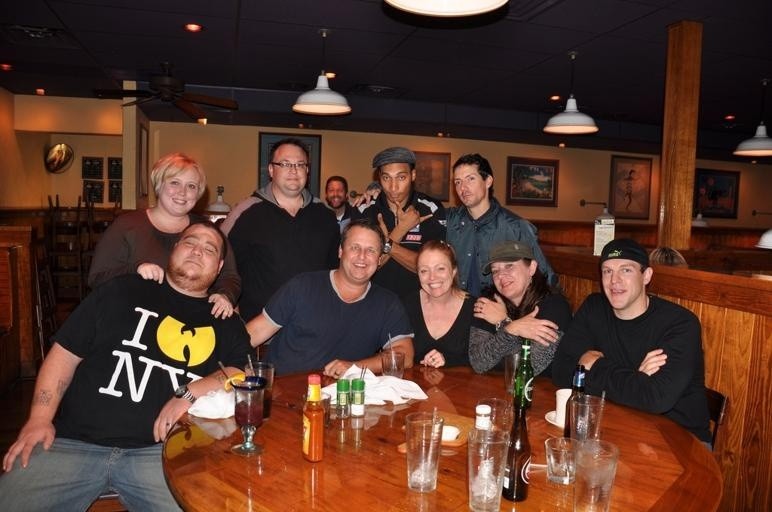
[271, 161, 308, 169]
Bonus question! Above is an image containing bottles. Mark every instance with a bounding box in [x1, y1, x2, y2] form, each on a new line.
[303, 372, 324, 463]
[336, 379, 351, 418]
[351, 378, 366, 418]
[502, 395, 532, 502]
[513, 339, 535, 409]
[564, 364, 591, 443]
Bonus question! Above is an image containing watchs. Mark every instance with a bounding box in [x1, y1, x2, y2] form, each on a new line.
[382, 237, 395, 259]
[174, 383, 199, 405]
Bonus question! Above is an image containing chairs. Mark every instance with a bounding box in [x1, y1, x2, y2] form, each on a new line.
[704, 387, 728, 452]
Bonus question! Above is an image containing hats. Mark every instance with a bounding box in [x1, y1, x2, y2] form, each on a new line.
[372, 146, 416, 168]
[600, 237, 650, 267]
[482, 241, 534, 275]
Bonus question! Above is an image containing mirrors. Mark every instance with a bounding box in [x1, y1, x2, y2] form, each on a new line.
[45, 142, 75, 174]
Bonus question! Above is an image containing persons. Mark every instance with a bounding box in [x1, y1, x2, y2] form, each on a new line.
[552, 238, 711, 454]
[245, 219, 413, 375]
[399, 241, 476, 369]
[648, 244, 689, 269]
[473, 240, 572, 377]
[444, 153, 561, 300]
[361, 147, 444, 298]
[1, 219, 259, 512]
[321, 176, 362, 234]
[87, 152, 242, 321]
[221, 138, 341, 323]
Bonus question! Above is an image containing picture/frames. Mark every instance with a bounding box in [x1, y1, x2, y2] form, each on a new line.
[608, 154, 653, 220]
[258, 131, 323, 199]
[505, 156, 560, 208]
[412, 150, 452, 202]
[692, 167, 741, 219]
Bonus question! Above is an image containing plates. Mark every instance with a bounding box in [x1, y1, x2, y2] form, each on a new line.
[545, 411, 565, 430]
[400, 412, 475, 456]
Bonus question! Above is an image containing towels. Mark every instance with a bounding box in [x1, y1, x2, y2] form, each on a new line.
[187, 388, 237, 420]
[321, 363, 429, 406]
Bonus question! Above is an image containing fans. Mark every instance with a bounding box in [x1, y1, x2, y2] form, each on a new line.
[91, 60, 239, 121]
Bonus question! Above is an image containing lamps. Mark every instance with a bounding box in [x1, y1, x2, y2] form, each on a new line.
[292, 70, 352, 116]
[542, 94, 599, 135]
[733, 121, 772, 157]
[382, 0, 509, 18]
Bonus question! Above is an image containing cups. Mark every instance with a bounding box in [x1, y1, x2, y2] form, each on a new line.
[506, 353, 520, 391]
[304, 391, 331, 424]
[232, 378, 266, 456]
[545, 438, 573, 485]
[405, 412, 444, 492]
[477, 397, 508, 429]
[556, 388, 572, 423]
[380, 348, 405, 378]
[570, 396, 604, 440]
[469, 429, 510, 510]
[573, 440, 620, 512]
[246, 361, 275, 395]
